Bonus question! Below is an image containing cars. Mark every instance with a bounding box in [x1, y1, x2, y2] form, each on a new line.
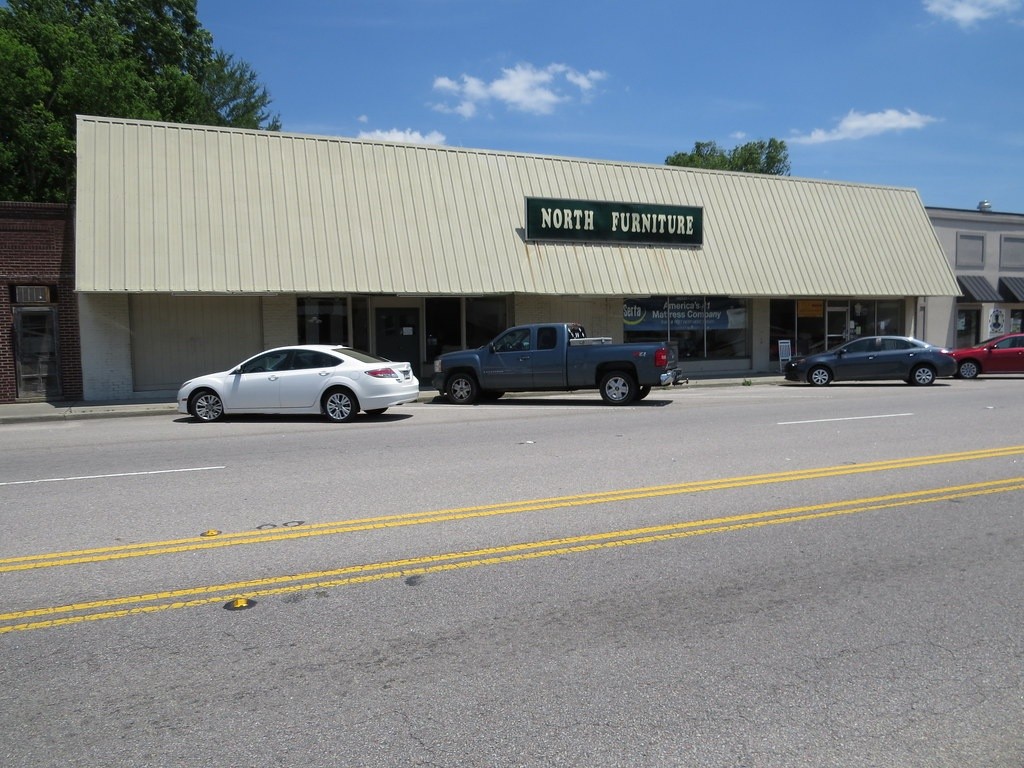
[178, 345, 420, 422]
[952, 333, 1024, 379]
[784, 335, 958, 388]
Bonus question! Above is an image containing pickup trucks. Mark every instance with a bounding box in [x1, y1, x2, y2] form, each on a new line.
[431, 322, 688, 405]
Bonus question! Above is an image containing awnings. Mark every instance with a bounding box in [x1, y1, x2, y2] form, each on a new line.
[956, 276, 1003, 303]
[998, 277, 1024, 302]
